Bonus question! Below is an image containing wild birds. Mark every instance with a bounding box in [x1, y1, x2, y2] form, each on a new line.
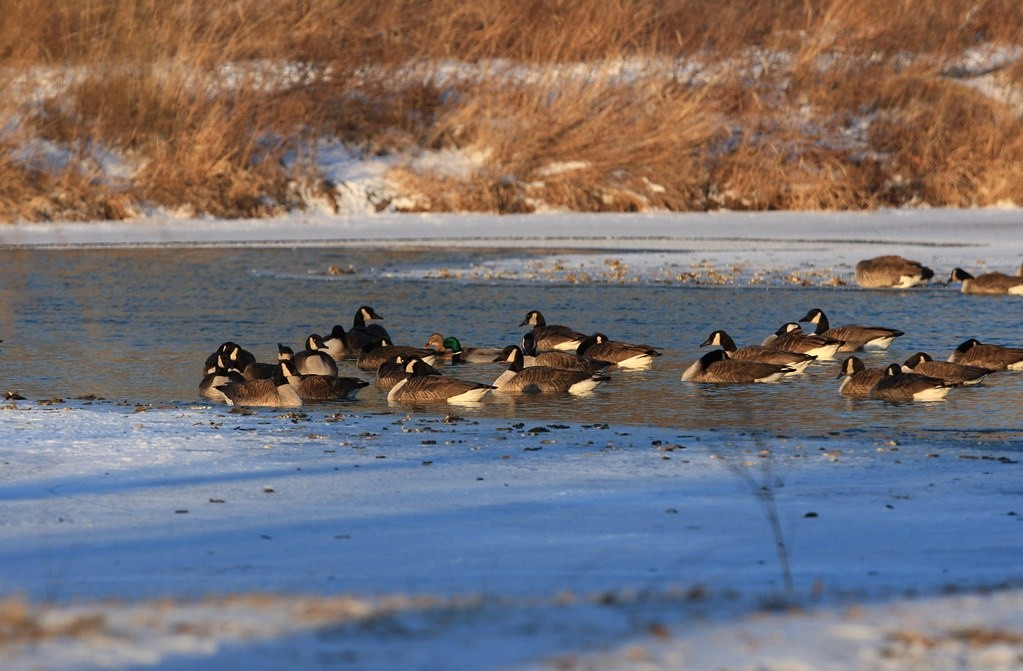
[198, 305, 1022, 421]
[855, 255, 1023, 297]
